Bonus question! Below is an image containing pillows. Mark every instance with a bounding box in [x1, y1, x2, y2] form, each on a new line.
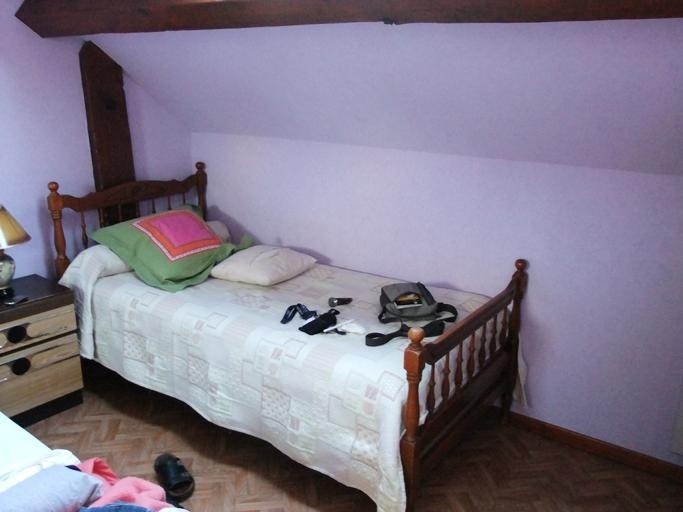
[84, 220, 230, 272]
[83, 204, 255, 295]
[211, 243, 319, 284]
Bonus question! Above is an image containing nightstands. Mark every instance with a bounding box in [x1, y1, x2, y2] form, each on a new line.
[0, 274, 84, 427]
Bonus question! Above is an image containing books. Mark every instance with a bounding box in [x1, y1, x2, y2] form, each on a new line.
[392, 291, 423, 309]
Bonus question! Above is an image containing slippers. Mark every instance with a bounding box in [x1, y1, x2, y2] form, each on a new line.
[153, 447, 195, 500]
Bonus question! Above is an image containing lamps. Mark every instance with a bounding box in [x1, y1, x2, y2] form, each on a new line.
[0, 204, 32, 294]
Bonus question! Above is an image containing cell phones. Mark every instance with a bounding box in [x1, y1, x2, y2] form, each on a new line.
[4, 295, 27, 306]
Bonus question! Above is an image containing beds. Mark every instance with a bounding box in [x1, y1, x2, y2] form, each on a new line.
[0, 411, 191, 512]
[47, 161, 527, 512]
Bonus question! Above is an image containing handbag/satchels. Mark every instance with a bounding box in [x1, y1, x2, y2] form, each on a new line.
[378, 281, 444, 318]
